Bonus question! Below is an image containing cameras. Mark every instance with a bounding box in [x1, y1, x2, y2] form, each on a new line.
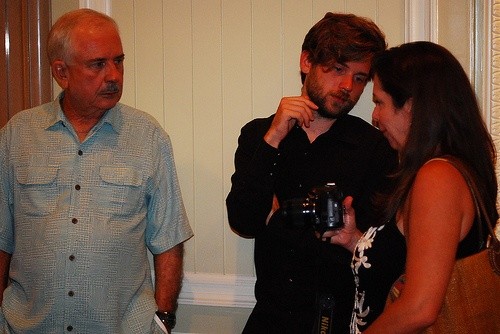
[297, 181, 346, 232]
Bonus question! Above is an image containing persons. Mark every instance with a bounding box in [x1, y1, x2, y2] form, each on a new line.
[316, 41, 500, 334]
[0, 8, 194, 334]
[226, 11, 400, 334]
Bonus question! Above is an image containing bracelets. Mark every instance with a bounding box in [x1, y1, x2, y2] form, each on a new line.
[156, 312, 176, 329]
